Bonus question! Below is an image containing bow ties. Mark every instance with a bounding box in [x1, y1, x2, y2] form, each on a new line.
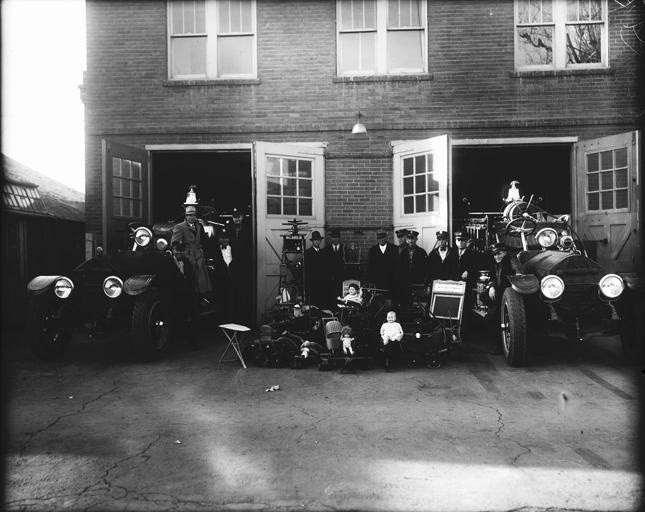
[441, 248, 446, 251]
[222, 246, 226, 249]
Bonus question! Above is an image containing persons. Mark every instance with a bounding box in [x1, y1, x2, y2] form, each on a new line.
[166, 206, 483, 373]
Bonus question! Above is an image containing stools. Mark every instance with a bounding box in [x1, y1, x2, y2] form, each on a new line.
[217, 323, 251, 369]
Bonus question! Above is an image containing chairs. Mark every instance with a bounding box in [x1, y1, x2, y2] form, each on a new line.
[339, 279, 363, 324]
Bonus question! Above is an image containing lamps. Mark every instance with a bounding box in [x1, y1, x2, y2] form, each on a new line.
[352, 110, 367, 135]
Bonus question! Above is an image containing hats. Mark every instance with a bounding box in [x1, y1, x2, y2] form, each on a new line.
[407, 230, 419, 236]
[233, 207, 244, 215]
[376, 232, 386, 238]
[310, 231, 324, 241]
[185, 206, 197, 215]
[331, 230, 340, 238]
[396, 229, 409, 235]
[454, 232, 467, 239]
[436, 231, 449, 240]
[489, 242, 505, 254]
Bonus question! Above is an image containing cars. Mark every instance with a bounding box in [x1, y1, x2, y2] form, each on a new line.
[452, 194, 645, 367]
[24, 203, 254, 364]
